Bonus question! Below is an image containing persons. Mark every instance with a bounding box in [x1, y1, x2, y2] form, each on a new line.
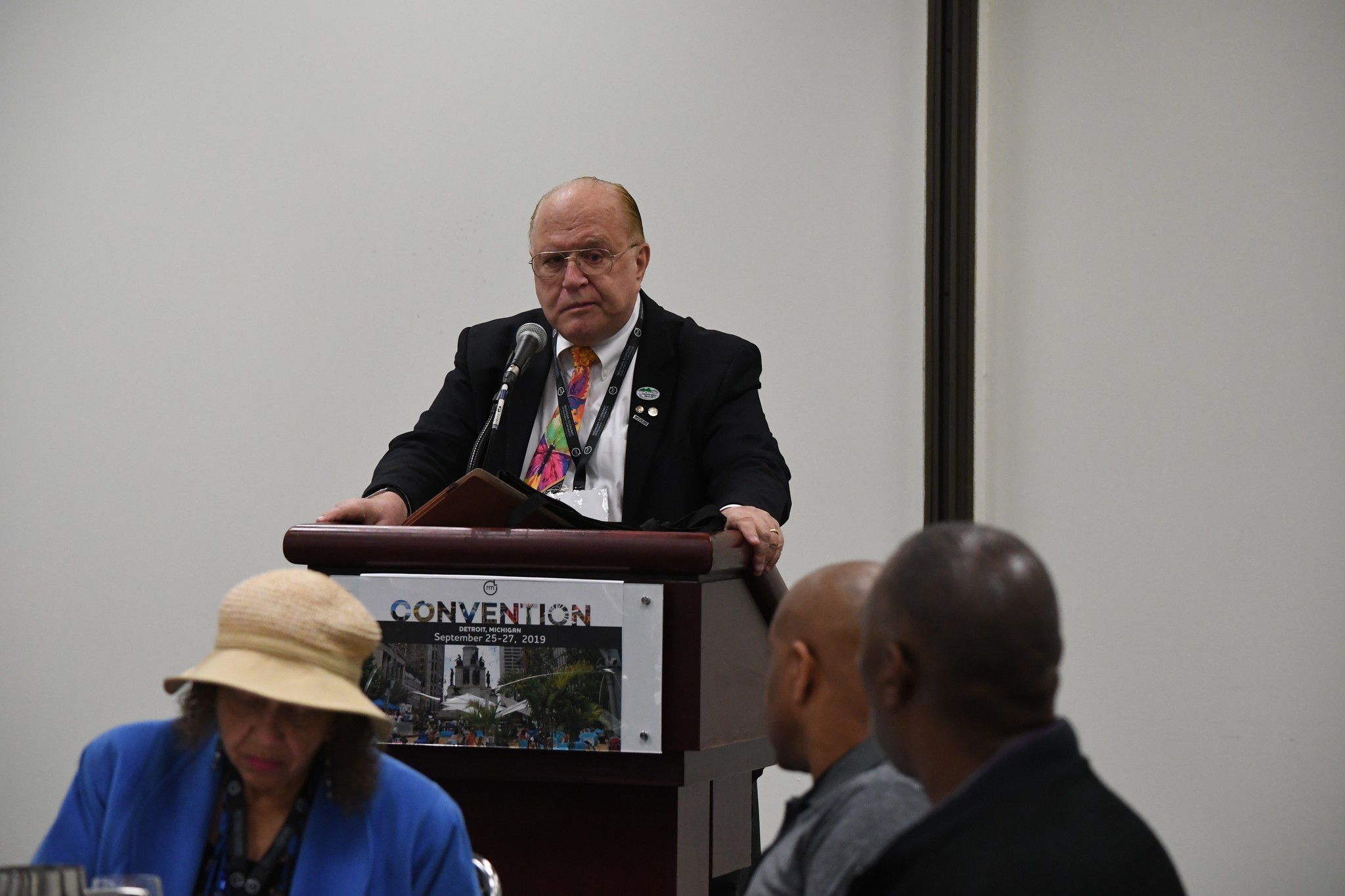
[454, 654, 462, 666]
[31, 565, 485, 896]
[446, 710, 621, 752]
[373, 710, 439, 746]
[479, 656, 485, 668]
[312, 176, 793, 577]
[745, 560, 933, 896]
[486, 671, 490, 688]
[840, 521, 1186, 896]
[449, 667, 454, 685]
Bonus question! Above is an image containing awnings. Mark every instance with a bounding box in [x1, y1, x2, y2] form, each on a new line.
[372, 698, 400, 710]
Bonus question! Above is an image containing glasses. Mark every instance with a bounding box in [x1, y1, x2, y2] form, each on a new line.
[529, 243, 641, 280]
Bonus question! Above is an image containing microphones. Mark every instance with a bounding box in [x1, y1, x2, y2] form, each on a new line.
[502, 322, 548, 388]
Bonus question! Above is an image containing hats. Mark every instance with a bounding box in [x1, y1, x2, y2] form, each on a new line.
[164, 569, 395, 739]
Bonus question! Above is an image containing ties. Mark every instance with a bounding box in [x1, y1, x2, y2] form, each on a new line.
[522, 344, 602, 496]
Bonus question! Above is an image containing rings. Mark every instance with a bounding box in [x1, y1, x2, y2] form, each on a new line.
[770, 529, 779, 535]
